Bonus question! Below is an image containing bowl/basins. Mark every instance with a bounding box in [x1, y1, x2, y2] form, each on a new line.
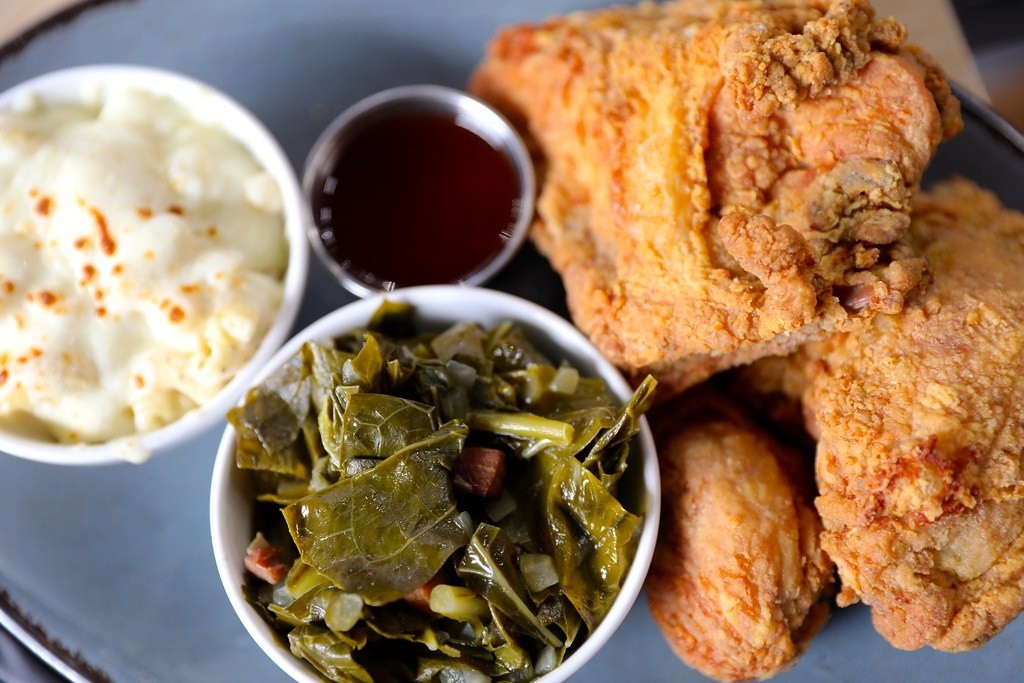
[302, 85, 536, 299]
[208, 283, 662, 683]
[0, 62, 311, 465]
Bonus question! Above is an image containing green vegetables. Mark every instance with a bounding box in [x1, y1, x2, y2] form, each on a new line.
[222, 300, 659, 683]
